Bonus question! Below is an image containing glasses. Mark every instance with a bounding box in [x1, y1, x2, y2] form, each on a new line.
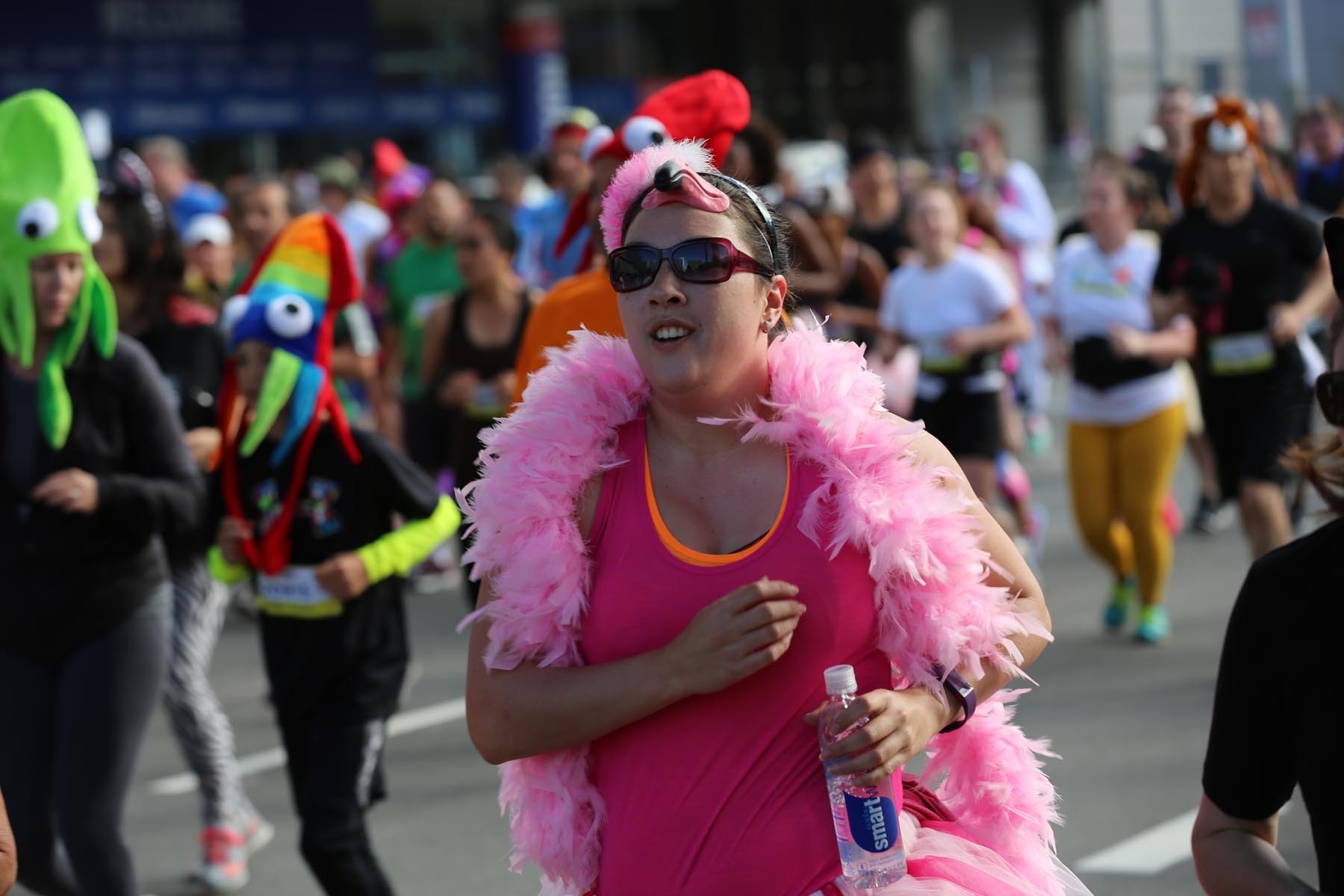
[610, 237, 781, 292]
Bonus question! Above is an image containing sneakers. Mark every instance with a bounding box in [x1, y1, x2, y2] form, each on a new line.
[184, 798, 275, 892]
[1103, 577, 1135, 629]
[1125, 601, 1173, 650]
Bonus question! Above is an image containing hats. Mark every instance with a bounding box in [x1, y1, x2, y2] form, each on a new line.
[553, 106, 600, 132]
[179, 213, 233, 247]
[209, 207, 368, 461]
[1, 90, 119, 449]
[557, 67, 747, 273]
[317, 160, 359, 195]
[1177, 97, 1270, 209]
[774, 138, 853, 216]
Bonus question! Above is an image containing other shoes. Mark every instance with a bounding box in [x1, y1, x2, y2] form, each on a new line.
[1023, 423, 1052, 459]
[1198, 496, 1234, 537]
[1013, 505, 1049, 553]
[413, 562, 460, 594]
[1160, 502, 1183, 535]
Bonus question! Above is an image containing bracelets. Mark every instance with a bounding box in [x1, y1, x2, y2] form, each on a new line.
[929, 655, 979, 734]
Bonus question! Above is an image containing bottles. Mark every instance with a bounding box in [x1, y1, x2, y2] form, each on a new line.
[822, 661, 909, 891]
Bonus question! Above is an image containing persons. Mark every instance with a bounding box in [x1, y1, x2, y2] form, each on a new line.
[465, 138, 1089, 896]
[0, 71, 1343, 896]
[199, 210, 461, 896]
[1190, 220, 1344, 896]
